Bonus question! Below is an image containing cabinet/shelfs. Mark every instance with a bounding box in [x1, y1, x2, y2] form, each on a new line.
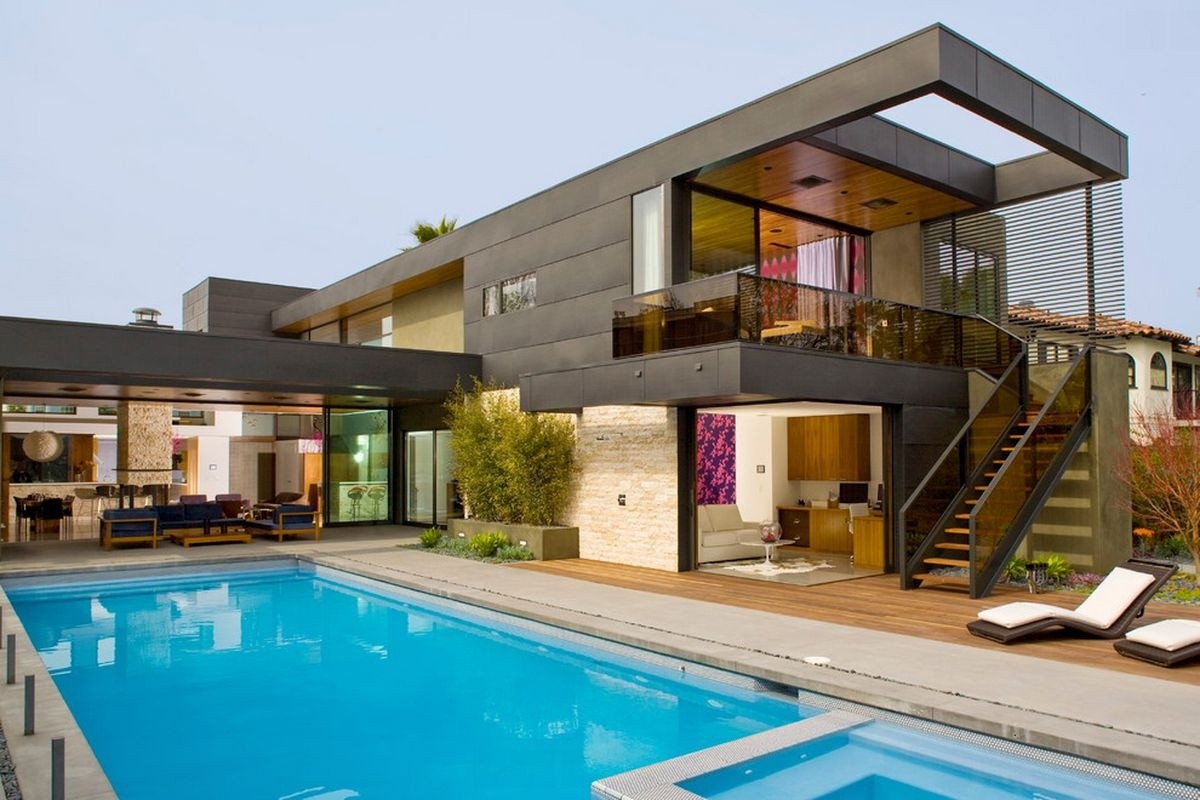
[777, 505, 886, 568]
[787, 414, 872, 482]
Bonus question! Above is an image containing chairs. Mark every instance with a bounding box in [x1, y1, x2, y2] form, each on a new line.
[217, 495, 242, 504]
[14, 495, 75, 540]
[181, 496, 206, 504]
[967, 558, 1200, 668]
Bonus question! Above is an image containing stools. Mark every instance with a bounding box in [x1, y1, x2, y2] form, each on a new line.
[76, 484, 161, 516]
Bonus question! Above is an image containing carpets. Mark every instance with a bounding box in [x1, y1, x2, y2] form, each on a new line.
[698, 554, 885, 587]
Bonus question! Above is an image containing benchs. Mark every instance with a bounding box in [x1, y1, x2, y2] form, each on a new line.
[247, 506, 320, 542]
[99, 510, 161, 549]
[157, 504, 246, 537]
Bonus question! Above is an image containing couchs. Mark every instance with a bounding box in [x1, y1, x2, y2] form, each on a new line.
[696, 504, 775, 563]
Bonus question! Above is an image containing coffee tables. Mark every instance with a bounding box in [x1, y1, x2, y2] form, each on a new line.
[742, 540, 795, 563]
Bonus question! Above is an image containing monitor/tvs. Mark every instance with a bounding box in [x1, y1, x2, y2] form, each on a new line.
[839, 483, 868, 509]
[876, 483, 883, 504]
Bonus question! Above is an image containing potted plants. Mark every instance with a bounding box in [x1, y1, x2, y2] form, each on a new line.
[444, 375, 579, 561]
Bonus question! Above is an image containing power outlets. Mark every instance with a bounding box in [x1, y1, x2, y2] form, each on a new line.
[757, 465, 765, 473]
